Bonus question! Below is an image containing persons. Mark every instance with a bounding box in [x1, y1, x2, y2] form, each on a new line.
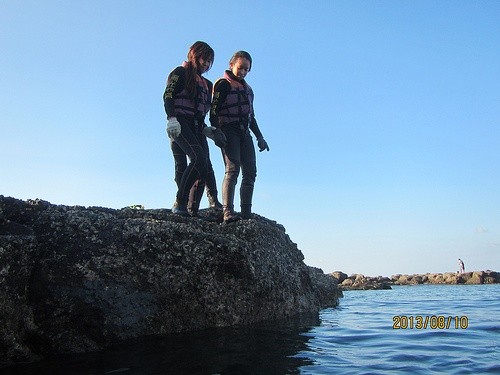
[457, 258, 465, 273]
[209, 50, 270, 225]
[187, 137, 223, 214]
[163, 41, 217, 216]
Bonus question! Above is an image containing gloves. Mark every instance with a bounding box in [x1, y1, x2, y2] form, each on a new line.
[216, 133, 227, 149]
[258, 138, 269, 152]
[203, 126, 219, 141]
[167, 117, 181, 139]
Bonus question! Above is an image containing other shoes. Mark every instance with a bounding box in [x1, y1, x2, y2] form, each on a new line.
[223, 213, 239, 221]
[171, 199, 189, 217]
[209, 199, 222, 211]
